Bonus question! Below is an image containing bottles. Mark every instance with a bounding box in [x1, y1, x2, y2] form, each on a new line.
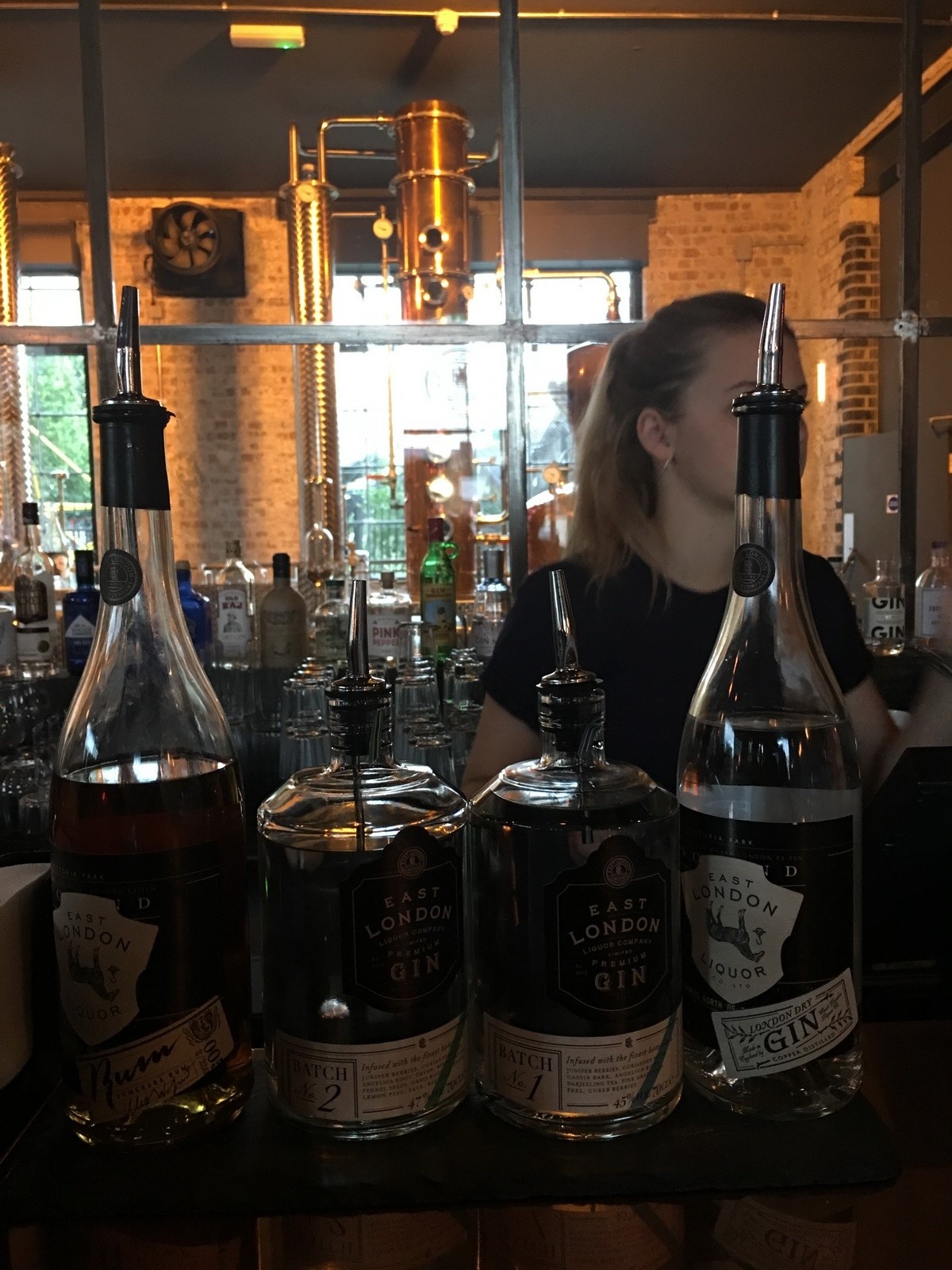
[0, 275, 952, 1270]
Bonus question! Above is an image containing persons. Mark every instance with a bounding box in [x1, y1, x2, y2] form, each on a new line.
[460, 291, 888, 798]
